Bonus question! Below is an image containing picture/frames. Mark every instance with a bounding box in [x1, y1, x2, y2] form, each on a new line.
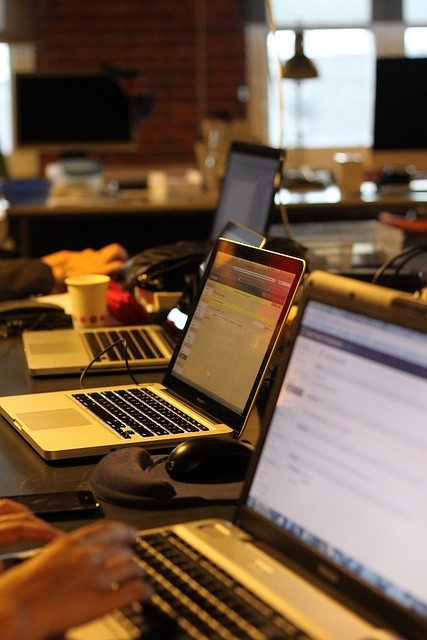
[5, 173, 427, 263]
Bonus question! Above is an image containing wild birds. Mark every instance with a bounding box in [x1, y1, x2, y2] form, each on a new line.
[20, 221, 268, 376]
[1, 270, 427, 640]
[1, 238, 307, 462]
[204, 137, 288, 243]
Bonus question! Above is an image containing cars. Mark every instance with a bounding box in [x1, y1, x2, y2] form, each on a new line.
[56, 267, 126, 331]
[332, 148, 377, 203]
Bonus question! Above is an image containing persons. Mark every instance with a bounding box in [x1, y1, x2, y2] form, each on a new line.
[0, 242, 128, 300]
[0, 497, 152, 639]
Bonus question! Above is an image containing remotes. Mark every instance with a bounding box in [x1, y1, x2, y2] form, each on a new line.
[166, 435, 258, 485]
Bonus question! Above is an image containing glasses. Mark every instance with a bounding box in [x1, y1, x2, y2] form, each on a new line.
[281, 29, 318, 80]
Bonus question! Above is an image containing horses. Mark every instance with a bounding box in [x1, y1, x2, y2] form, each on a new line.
[1, 489, 105, 521]
